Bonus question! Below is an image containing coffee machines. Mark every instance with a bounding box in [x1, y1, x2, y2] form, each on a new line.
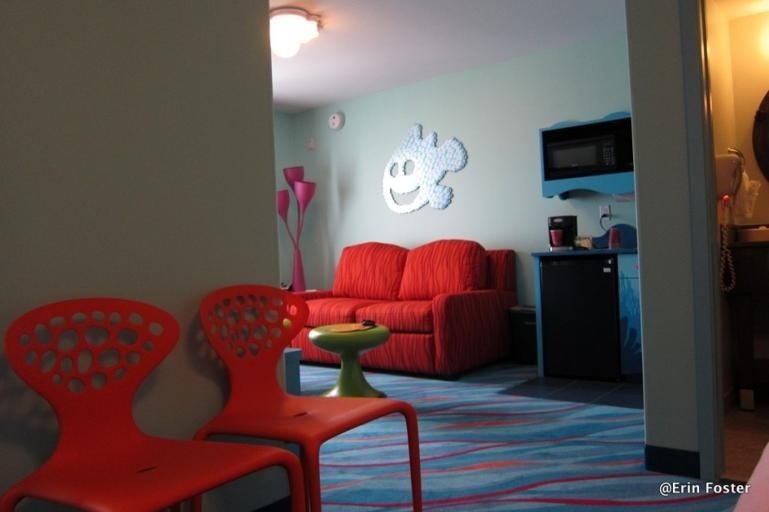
[548, 216, 577, 251]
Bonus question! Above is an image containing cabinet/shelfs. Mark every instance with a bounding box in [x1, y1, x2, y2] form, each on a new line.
[532, 251, 641, 381]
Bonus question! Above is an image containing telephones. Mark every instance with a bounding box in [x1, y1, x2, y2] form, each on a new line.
[714, 146, 744, 209]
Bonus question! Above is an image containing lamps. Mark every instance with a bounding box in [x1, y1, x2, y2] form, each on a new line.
[276, 165, 315, 291]
[269, 7, 323, 56]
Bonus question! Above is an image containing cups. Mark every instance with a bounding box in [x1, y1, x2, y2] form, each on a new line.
[608, 228, 620, 248]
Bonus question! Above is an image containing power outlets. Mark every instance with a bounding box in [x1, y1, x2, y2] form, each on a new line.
[599, 204, 611, 222]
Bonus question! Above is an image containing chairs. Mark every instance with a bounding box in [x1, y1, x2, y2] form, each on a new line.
[0, 296, 307, 512]
[191, 284, 423, 512]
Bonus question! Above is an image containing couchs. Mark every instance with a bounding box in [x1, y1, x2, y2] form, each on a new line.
[287, 240, 515, 372]
